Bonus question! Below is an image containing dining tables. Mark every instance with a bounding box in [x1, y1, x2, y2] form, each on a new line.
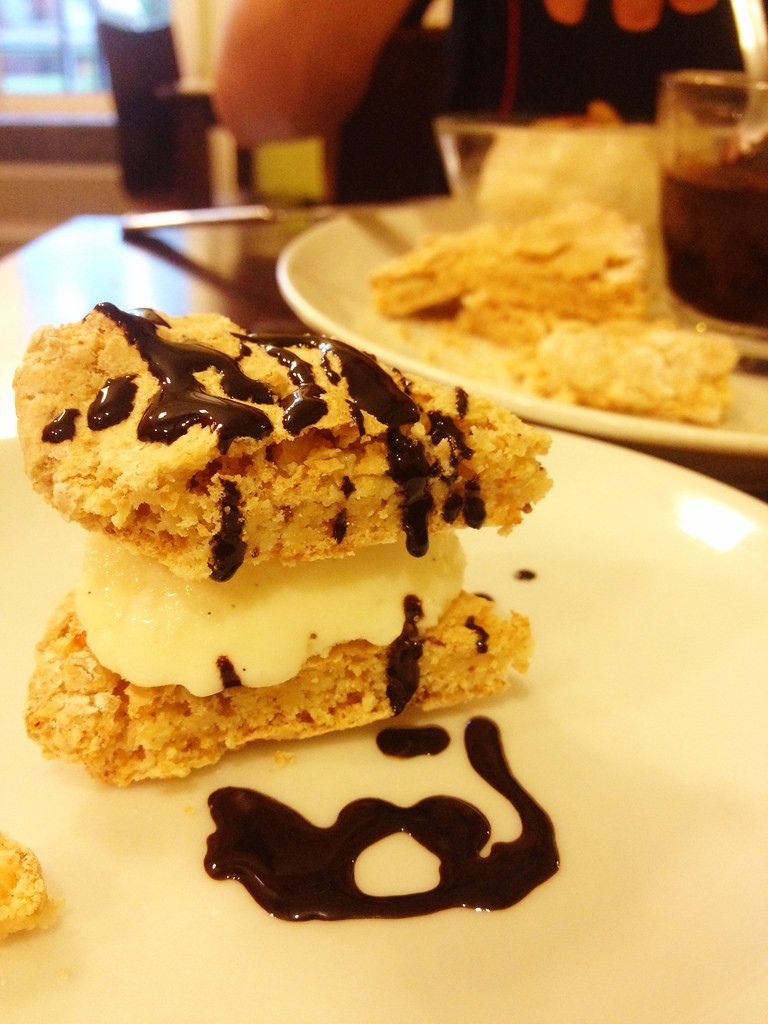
[0, 201, 768, 508]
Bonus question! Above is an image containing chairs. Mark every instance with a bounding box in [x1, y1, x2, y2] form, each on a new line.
[92, 20, 218, 201]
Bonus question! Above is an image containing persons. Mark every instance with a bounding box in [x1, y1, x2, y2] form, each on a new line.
[211, 0, 767, 201]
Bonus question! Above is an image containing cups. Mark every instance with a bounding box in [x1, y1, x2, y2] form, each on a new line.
[653, 67, 768, 341]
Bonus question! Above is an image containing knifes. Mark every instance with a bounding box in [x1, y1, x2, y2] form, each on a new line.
[122, 201, 395, 229]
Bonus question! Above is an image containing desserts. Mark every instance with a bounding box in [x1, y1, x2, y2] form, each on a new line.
[7, 300, 555, 785]
[0, 831, 63, 942]
[364, 201, 740, 427]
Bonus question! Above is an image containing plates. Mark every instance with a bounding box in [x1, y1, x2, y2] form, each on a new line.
[0, 385, 766, 1022]
[274, 195, 768, 464]
